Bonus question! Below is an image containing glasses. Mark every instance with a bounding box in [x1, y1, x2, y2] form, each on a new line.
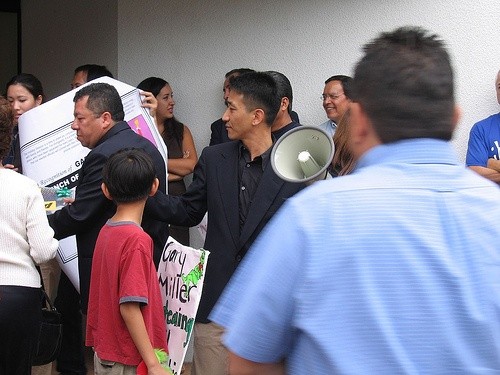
[319, 91, 344, 100]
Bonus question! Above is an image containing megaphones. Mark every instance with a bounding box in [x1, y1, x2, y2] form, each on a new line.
[270, 125, 335, 186]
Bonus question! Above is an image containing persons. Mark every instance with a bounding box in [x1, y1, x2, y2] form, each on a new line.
[0, 64, 500, 375]
[207, 24, 500, 375]
